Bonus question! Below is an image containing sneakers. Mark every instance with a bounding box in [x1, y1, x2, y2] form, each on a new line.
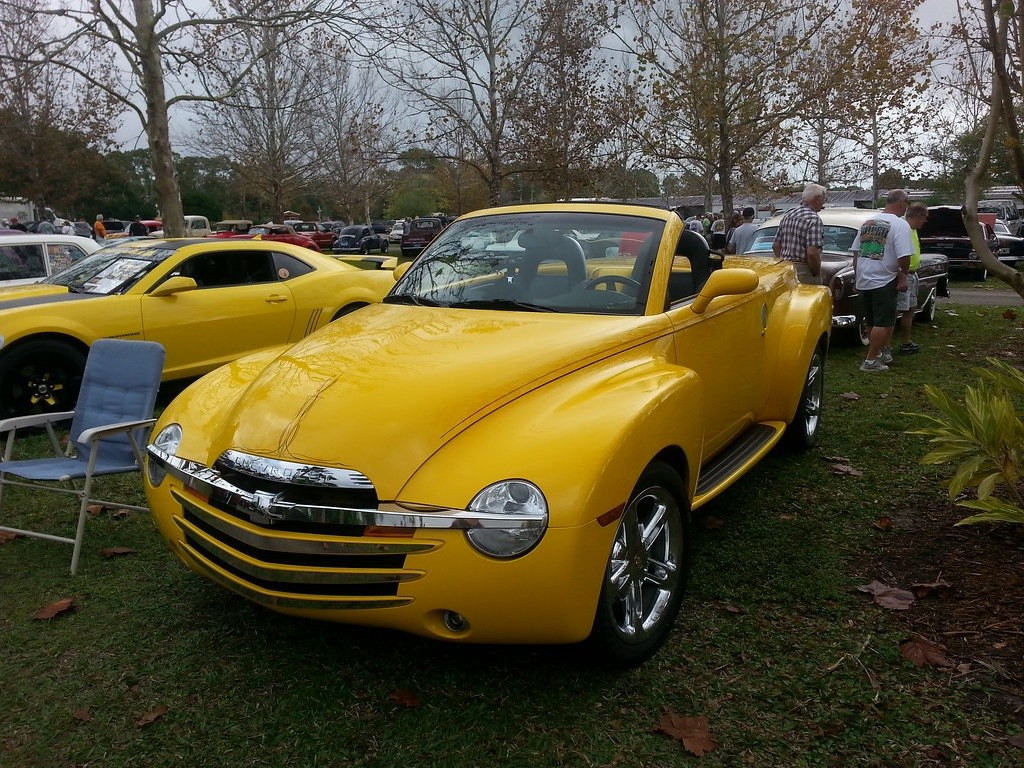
[900, 341, 920, 353]
[876, 353, 893, 365]
[859, 358, 889, 374]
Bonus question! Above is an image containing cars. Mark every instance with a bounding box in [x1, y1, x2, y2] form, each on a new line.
[70, 222, 92, 238]
[147, 215, 212, 238]
[993, 219, 1024, 268]
[0, 234, 102, 287]
[741, 207, 949, 346]
[386, 220, 405, 243]
[205, 219, 338, 253]
[400, 218, 443, 256]
[0, 227, 71, 272]
[419, 216, 458, 229]
[146, 203, 835, 671]
[977, 199, 1023, 238]
[139, 220, 162, 234]
[914, 206, 999, 282]
[102, 221, 125, 235]
[0, 237, 399, 436]
[333, 223, 389, 255]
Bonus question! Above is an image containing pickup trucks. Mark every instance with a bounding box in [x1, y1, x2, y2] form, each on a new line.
[751, 210, 787, 227]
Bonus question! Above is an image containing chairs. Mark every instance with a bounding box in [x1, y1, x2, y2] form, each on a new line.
[512, 235, 586, 299]
[627, 228, 725, 312]
[0, 338, 165, 575]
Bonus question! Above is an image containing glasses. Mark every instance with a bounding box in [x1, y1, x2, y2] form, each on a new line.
[922, 213, 929, 221]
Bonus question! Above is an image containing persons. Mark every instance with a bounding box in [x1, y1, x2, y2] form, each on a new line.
[772, 183, 828, 285]
[128, 214, 149, 236]
[62, 221, 76, 235]
[685, 209, 745, 252]
[849, 188, 916, 372]
[896, 202, 930, 351]
[37, 216, 56, 235]
[94, 213, 107, 241]
[711, 214, 726, 250]
[0, 217, 28, 233]
[729, 207, 759, 255]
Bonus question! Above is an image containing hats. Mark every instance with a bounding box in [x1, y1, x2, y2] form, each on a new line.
[10, 217, 17, 222]
[743, 207, 754, 217]
[136, 215, 140, 219]
[3, 218, 9, 225]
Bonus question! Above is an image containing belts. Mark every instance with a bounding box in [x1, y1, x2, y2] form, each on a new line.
[908, 271, 915, 275]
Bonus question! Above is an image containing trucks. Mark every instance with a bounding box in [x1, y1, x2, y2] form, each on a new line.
[0, 196, 74, 231]
